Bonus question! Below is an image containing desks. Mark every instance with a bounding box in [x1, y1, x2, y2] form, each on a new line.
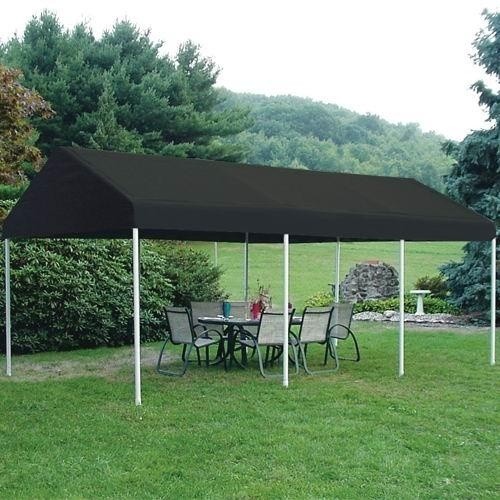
[410, 289, 431, 316]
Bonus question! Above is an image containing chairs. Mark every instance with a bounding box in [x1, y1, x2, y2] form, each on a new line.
[157, 302, 360, 378]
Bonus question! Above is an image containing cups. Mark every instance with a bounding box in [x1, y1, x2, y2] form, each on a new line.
[222, 301, 231, 318]
